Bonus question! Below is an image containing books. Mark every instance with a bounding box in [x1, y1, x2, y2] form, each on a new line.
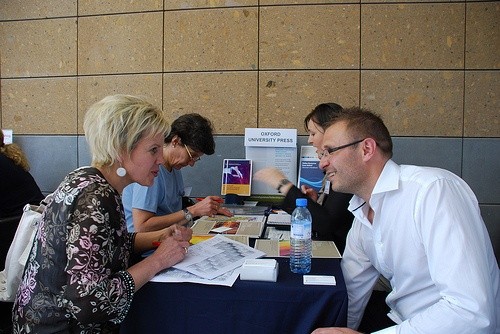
[221, 159, 252, 196]
[298, 147, 330, 195]
[245, 128, 297, 196]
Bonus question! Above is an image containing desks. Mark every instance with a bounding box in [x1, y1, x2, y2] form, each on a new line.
[133, 202, 349, 334]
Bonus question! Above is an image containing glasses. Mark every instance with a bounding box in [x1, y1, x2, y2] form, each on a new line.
[181, 141, 200, 162]
[318, 138, 378, 160]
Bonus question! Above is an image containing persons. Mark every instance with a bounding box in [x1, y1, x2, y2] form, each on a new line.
[12, 94, 193, 334]
[312, 106, 500, 334]
[252, 102, 355, 255]
[0, 129, 46, 334]
[121, 113, 234, 263]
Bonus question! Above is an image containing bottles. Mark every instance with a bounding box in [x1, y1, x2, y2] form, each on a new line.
[289, 198, 312, 274]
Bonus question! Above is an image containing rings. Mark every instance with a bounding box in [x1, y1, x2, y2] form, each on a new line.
[216, 205, 217, 210]
[184, 247, 188, 253]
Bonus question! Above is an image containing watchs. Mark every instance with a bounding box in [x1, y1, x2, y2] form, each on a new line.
[183, 208, 192, 221]
[276, 179, 290, 193]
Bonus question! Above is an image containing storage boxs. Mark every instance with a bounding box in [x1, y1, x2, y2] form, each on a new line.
[241, 259, 279, 283]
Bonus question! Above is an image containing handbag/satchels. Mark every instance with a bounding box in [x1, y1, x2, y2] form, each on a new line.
[0, 166, 92, 303]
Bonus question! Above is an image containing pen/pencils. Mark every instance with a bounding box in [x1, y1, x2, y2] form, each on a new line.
[152, 242, 192, 246]
[196, 197, 223, 203]
